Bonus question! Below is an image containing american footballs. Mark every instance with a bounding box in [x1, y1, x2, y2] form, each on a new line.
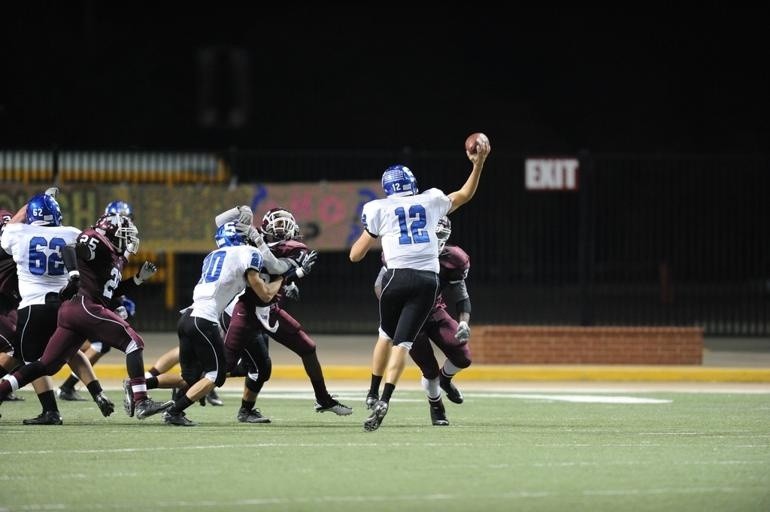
[466, 132, 489, 154]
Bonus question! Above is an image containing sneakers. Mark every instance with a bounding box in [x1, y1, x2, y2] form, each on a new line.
[441, 378, 463, 403]
[430, 400, 449, 425]
[364, 400, 389, 431]
[238, 406, 271, 423]
[314, 400, 353, 416]
[4, 379, 224, 426]
[366, 392, 379, 409]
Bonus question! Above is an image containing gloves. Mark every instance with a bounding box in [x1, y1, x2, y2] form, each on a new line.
[238, 205, 254, 226]
[136, 260, 158, 282]
[300, 249, 317, 275]
[284, 280, 300, 302]
[62, 275, 81, 300]
[454, 321, 471, 346]
[44, 187, 59, 198]
[236, 223, 260, 243]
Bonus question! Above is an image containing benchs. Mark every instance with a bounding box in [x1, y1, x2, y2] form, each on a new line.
[471, 326, 705, 365]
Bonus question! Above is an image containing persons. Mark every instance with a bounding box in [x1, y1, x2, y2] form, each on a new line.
[348, 142, 492, 431]
[363, 214, 472, 426]
[1, 186, 176, 426]
[119, 205, 350, 428]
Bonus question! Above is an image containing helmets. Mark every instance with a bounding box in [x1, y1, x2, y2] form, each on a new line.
[27, 193, 63, 226]
[435, 215, 452, 256]
[215, 219, 249, 248]
[260, 208, 300, 243]
[104, 200, 135, 221]
[96, 213, 139, 255]
[381, 164, 419, 196]
[0, 208, 15, 227]
